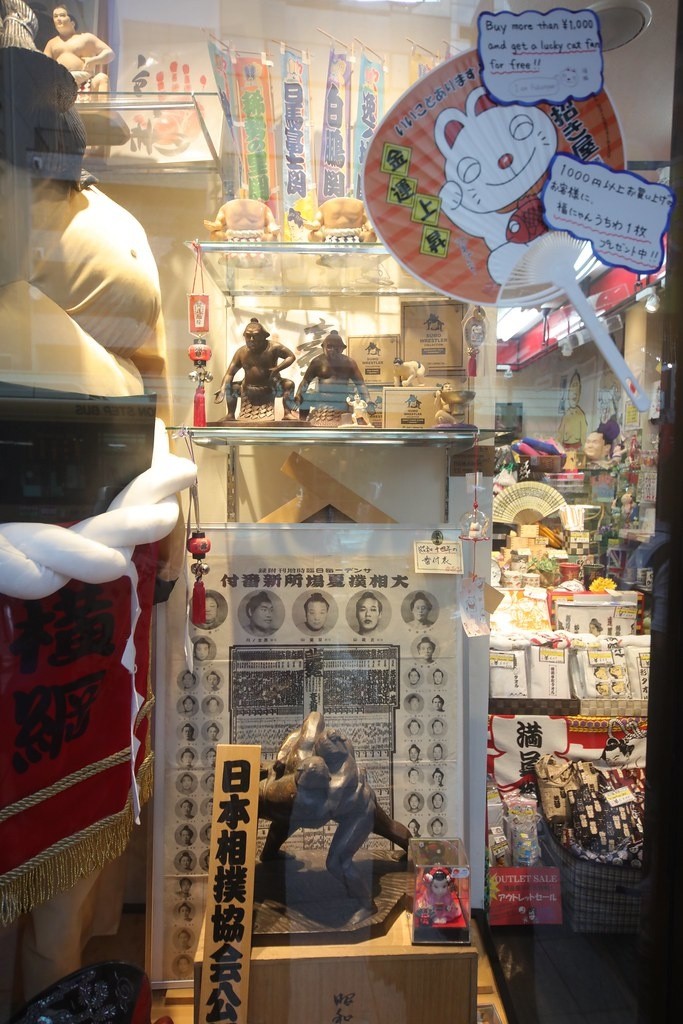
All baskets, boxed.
[519,453,567,473]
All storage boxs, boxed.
[487,839,564,936]
[542,821,643,937]
[517,454,562,473]
[496,523,594,577]
[547,586,645,637]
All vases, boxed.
[582,563,605,591]
[560,563,580,583]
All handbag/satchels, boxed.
[536,754,645,853]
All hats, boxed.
[0,46,87,182]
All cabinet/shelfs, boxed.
[160,241,516,450]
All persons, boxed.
[402,638,447,838]
[436,378,466,429]
[290,196,378,244]
[41,3,115,104]
[1,49,184,1019]
[393,358,428,387]
[418,869,463,926]
[298,593,330,635]
[243,588,279,638]
[584,419,644,526]
[177,635,221,975]
[406,590,434,629]
[203,194,279,258]
[295,331,374,427]
[214,317,292,421]
[355,591,383,635]
[199,593,220,625]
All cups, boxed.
[647,571,653,588]
[560,563,579,585]
[523,574,540,587]
[637,567,649,585]
[628,568,636,580]
[502,572,522,588]
[584,564,605,588]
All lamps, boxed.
[634,286,661,314]
[604,314,625,334]
[569,332,585,350]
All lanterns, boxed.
[188,339,212,367]
[186,532,211,559]
